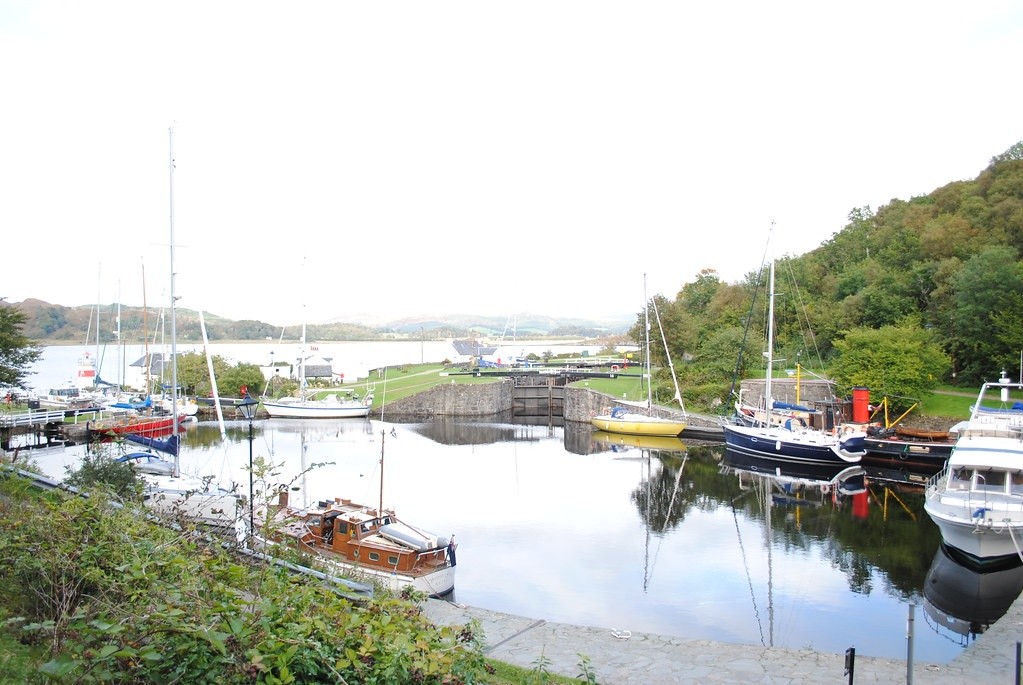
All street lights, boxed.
[235,390,257,551]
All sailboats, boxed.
[40,259,198,425]
[589,272,690,437]
[585,432,690,593]
[64,127,240,538]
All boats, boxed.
[920,542,1023,648]
[715,206,953,497]
[711,447,935,644]
[261,301,378,419]
[243,428,458,600]
[451,329,500,356]
[920,351,1023,563]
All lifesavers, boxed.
[95,422,103,429]
[769,417,776,424]
[899,451,909,461]
[843,426,855,435]
[900,467,908,475]
[819,485,830,494]
[777,418,783,425]
